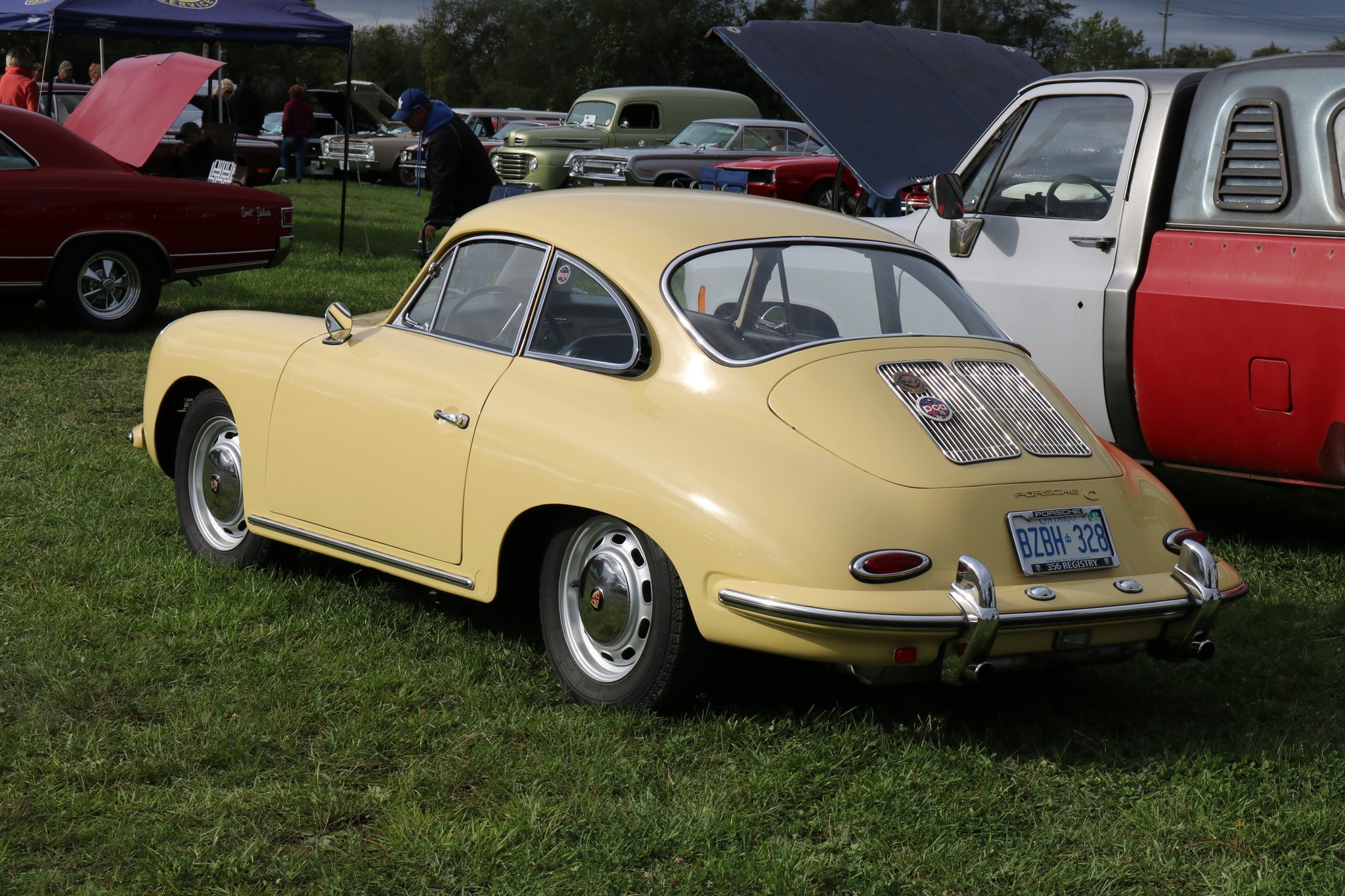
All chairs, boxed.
[672,166,750,194]
[411,186,534,269]
[556,333,650,371]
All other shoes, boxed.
[280,179,289,184]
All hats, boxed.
[391,88,430,122]
[31,63,43,71]
[59,60,73,70]
[175,121,199,140]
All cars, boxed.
[127,187,1250,710]
[0,51,296,337]
[561,119,931,217]
[34,79,570,191]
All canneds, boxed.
[418,239,428,252]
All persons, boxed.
[87,62,105,87]
[168,122,215,176]
[53,60,77,85]
[546,105,553,111]
[280,85,314,183]
[202,79,237,135]
[392,87,504,243]
[231,73,258,135]
[0,47,41,113]
[32,62,45,83]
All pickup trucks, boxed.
[682,20,1344,496]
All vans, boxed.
[489,87,790,190]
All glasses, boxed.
[223,89,233,95]
[179,133,193,142]
[37,69,43,72]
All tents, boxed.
[0,0,353,254]
